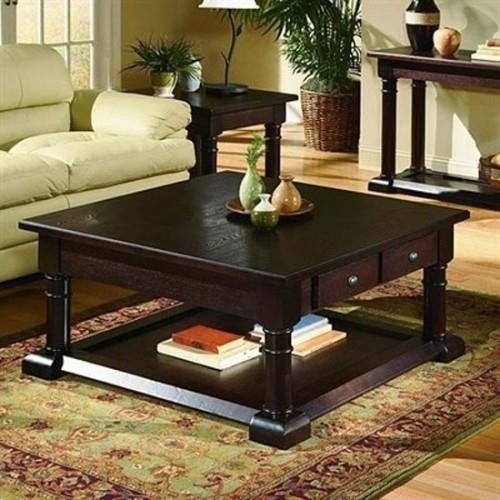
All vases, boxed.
[251,194,279,231]
[272,174,302,213]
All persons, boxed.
[291,339,346,361]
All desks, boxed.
[366,45,500,212]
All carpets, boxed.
[0,276,500,500]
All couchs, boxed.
[0,43,197,289]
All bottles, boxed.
[178,54,203,92]
[250,175,302,232]
[405,0,440,54]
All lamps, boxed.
[197,0,260,96]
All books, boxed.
[246,312,348,359]
[470,38,500,62]
[470,59,500,67]
[155,335,265,372]
[156,351,261,378]
[170,323,247,355]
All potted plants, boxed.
[239,135,269,211]
[117,32,207,97]
[215,0,362,153]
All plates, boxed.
[226,196,315,217]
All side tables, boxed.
[120,83,298,178]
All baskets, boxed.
[300,85,359,152]
[478,156,500,192]
